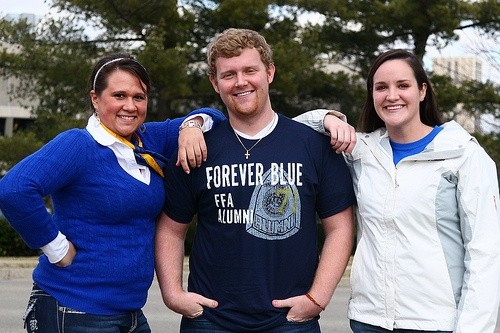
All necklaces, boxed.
[228,111,276,160]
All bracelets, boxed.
[178,120,202,133]
[305,293,325,312]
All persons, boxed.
[290,48,499,332]
[154,28,359,333]
[0,54,229,333]
